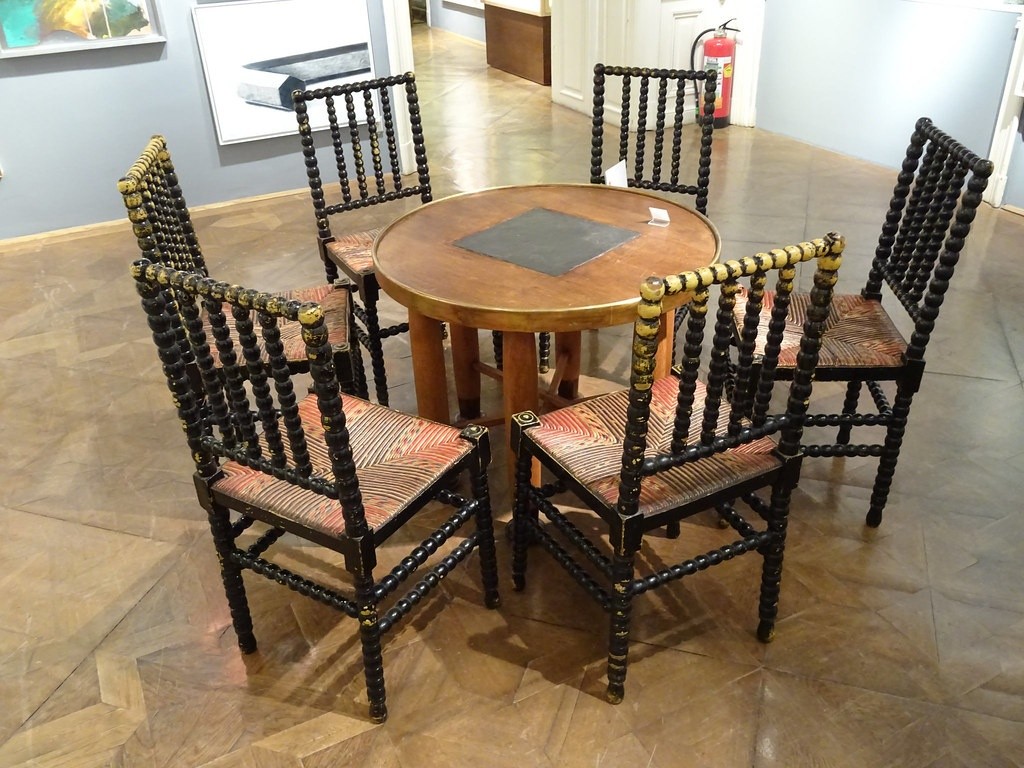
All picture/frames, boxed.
[191,0,380,146]
[0,0,167,59]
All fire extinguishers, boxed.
[689,17,741,130]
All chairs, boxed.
[507,232,844,705]
[132,258,500,724]
[719,119,994,528]
[539,64,718,373]
[293,71,505,410]
[118,136,370,420]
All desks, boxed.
[372,184,719,490]
